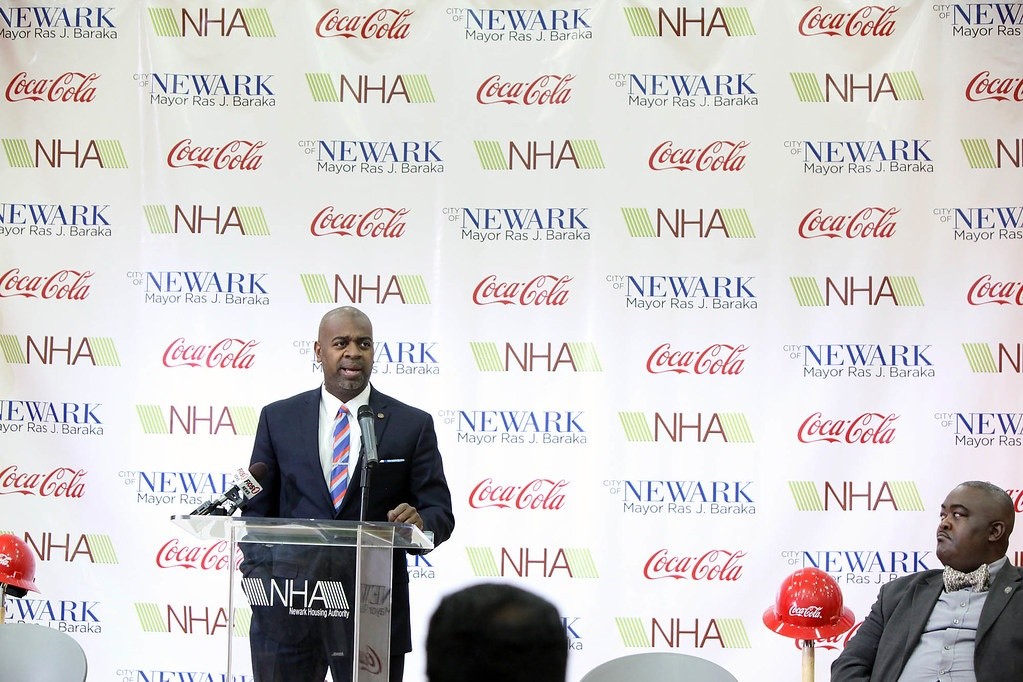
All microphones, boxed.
[357,404,378,467]
[190,463,269,515]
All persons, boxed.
[831,481,1023,682]
[425,583,568,682]
[237,307,455,682]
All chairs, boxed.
[0,622,88,682]
[581,652,738,682]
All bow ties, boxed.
[943,563,991,594]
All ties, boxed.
[329,405,350,512]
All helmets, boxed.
[0,534,42,598]
[762,567,856,640]
